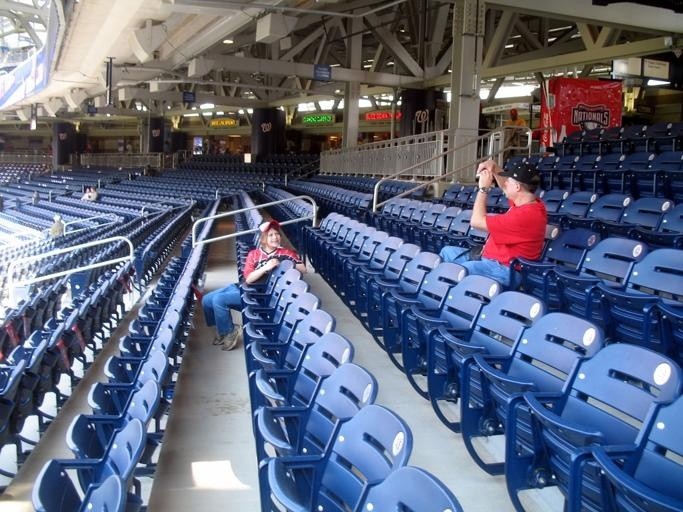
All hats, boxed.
[495,160,542,187]
[258,219,280,234]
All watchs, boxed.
[477,187,489,192]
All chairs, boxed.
[80,287,103,334]
[466,225,490,249]
[504,156,522,172]
[53,279,63,312]
[358,465,464,511]
[423,206,461,254]
[0,356,30,457]
[8,337,48,433]
[446,186,472,208]
[440,209,474,248]
[88,279,111,324]
[88,472,128,511]
[548,188,592,222]
[496,195,510,210]
[569,191,625,230]
[609,193,667,247]
[602,127,627,153]
[399,274,501,402]
[655,149,683,194]
[44,309,81,366]
[548,236,646,325]
[365,252,441,351]
[461,189,478,206]
[245,331,355,439]
[509,227,602,310]
[118,308,182,371]
[62,297,93,346]
[629,150,663,195]
[64,379,163,496]
[242,308,336,392]
[88,348,170,450]
[622,126,652,152]
[486,187,501,212]
[0,310,18,351]
[42,285,55,322]
[379,262,468,375]
[31,292,46,328]
[30,416,148,511]
[595,153,627,191]
[434,181,460,207]
[15,301,32,339]
[23,324,66,392]
[502,341,683,512]
[252,403,415,512]
[410,203,446,245]
[101,325,175,413]
[535,156,559,189]
[242,291,321,358]
[539,190,565,222]
[425,288,545,435]
[658,198,681,243]
[567,396,682,511]
[594,248,681,366]
[459,311,605,476]
[581,127,607,155]
[561,130,584,155]
[1,152,426,298]
[573,154,603,191]
[649,122,677,151]
[136,295,186,336]
[551,156,577,189]
[252,364,381,509]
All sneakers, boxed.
[212,333,226,346]
[222,322,244,352]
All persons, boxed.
[51,215,64,236]
[504,108,527,127]
[88,187,99,201]
[438,159,547,292]
[81,188,90,201]
[60,215,65,224]
[202,222,306,351]
